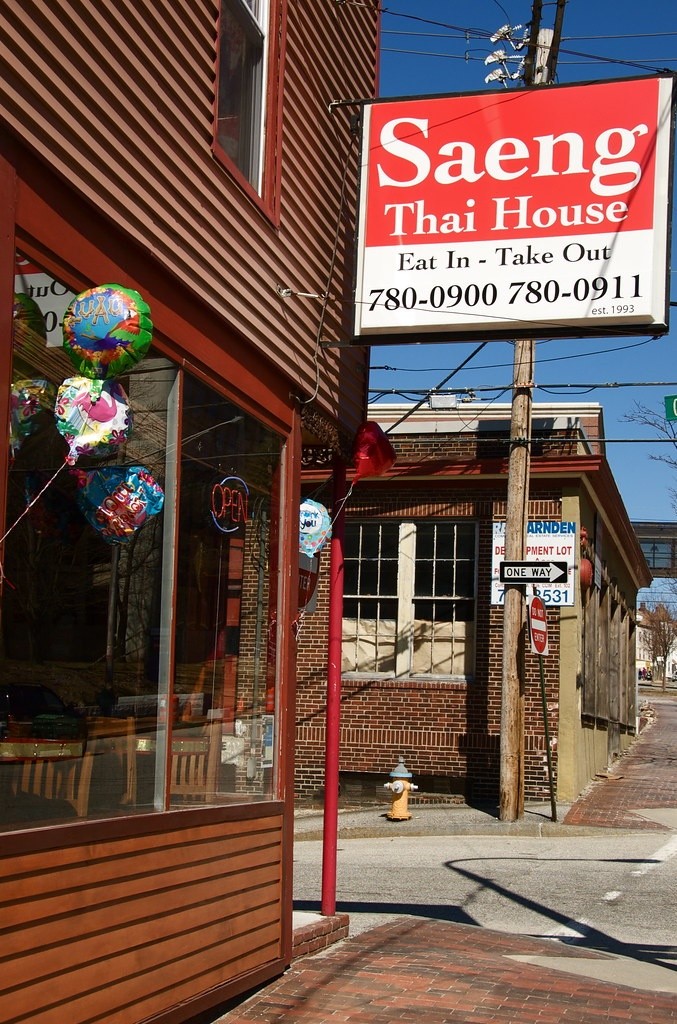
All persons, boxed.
[638,667,653,681]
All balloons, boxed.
[23,282,164,548]
[299,499,332,558]
[8,378,60,473]
[352,421,397,484]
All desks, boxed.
[105,737,207,756]
[0,736,82,762]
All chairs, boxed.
[169,718,222,810]
[83,715,154,819]
[6,717,98,821]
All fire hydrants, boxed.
[380,756,417,820]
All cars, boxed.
[672,671,677,682]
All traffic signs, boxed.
[500,560,571,586]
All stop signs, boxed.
[528,596,550,656]
